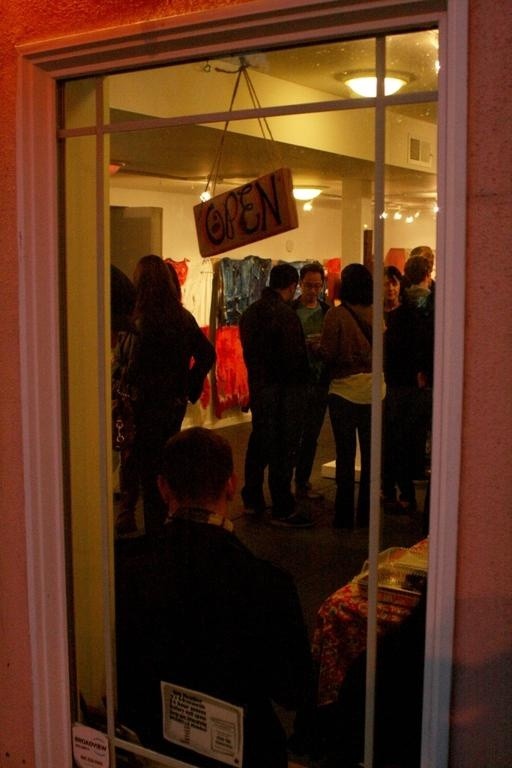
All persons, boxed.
[307,589,426,768]
[114,425,317,767]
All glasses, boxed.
[301,279,323,291]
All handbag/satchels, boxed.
[112,364,140,451]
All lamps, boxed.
[289,184,330,201]
[332,69,415,98]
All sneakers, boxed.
[329,514,369,533]
[268,507,323,529]
[383,500,420,516]
[294,483,327,499]
[243,503,269,518]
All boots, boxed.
[117,489,140,534]
